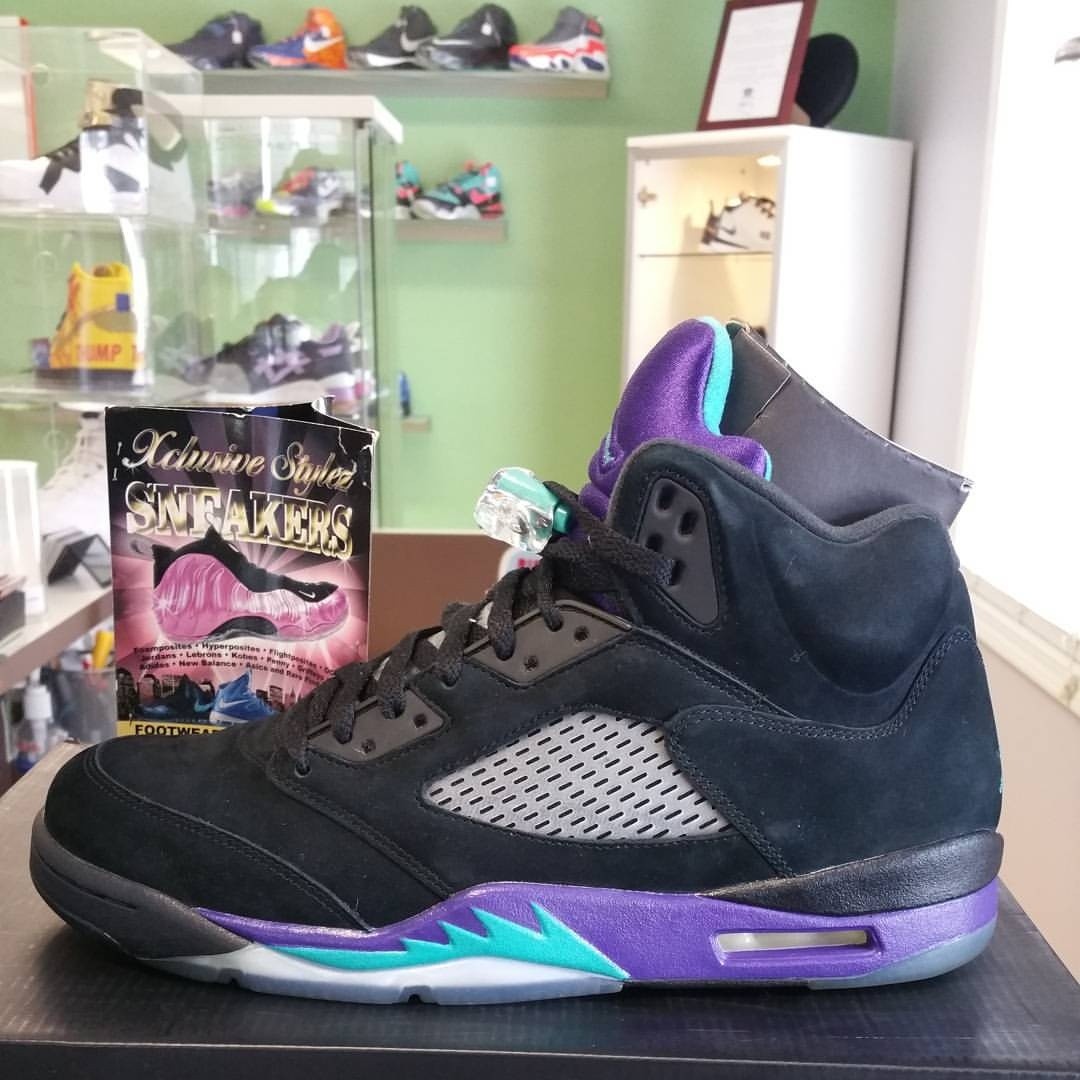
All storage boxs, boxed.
[0,738,1080,1080]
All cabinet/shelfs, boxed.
[146,69,615,246]
[620,125,914,441]
[0,24,405,431]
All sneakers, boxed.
[162,12,264,70]
[508,6,610,75]
[29,317,1005,1006]
[413,161,505,221]
[28,263,138,387]
[0,80,194,223]
[414,3,517,71]
[348,5,438,69]
[392,159,424,221]
[210,164,380,225]
[248,8,348,70]
[174,312,374,407]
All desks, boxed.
[0,575,113,696]
